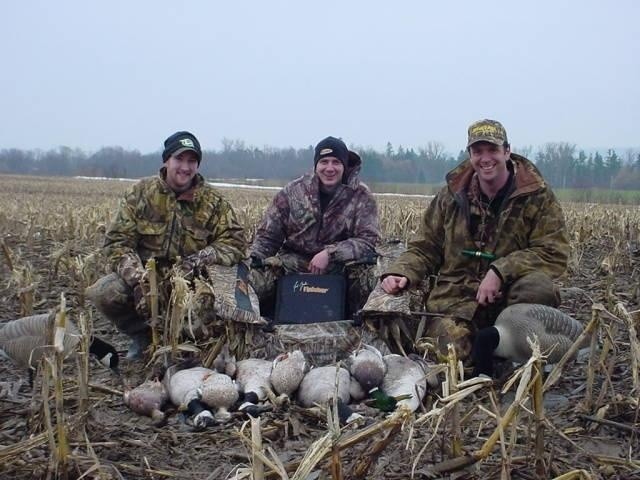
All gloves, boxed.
[182,259,195,282]
[132,283,150,316]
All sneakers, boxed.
[125,326,153,360]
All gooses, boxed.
[466,301,588,368]
[1,312,121,391]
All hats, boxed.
[465,118,508,149]
[313,135,349,172]
[162,131,202,167]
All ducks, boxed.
[120,341,440,431]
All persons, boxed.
[92,131,248,362]
[249,136,384,314]
[362,120,570,361]
